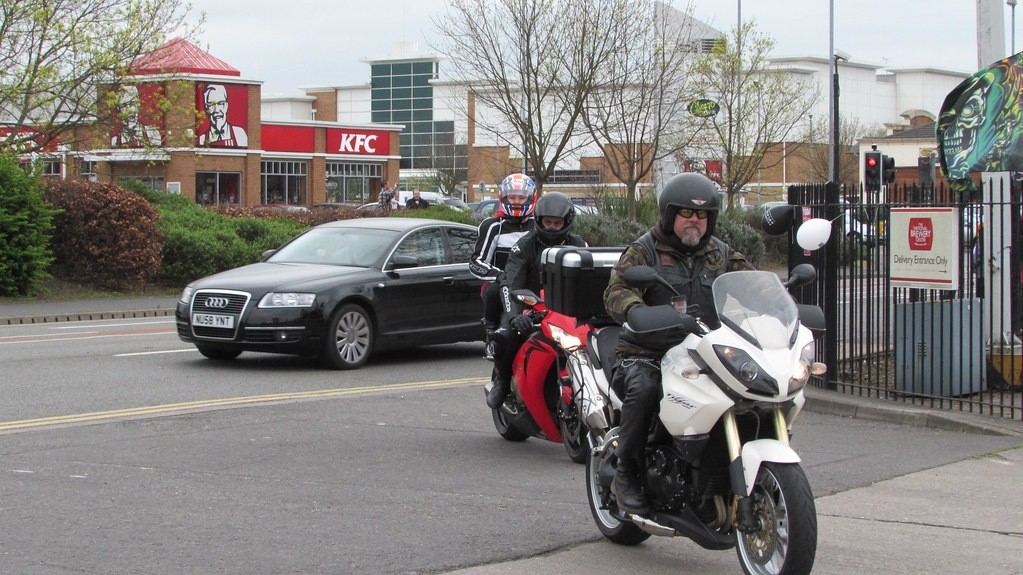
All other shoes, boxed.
[485,331,496,355]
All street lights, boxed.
[753,81,761,204]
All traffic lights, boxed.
[865,152,881,191]
[881,155,895,184]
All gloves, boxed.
[511,315,534,336]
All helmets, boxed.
[658,171,720,233]
[532,191,575,244]
[498,173,537,218]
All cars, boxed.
[839,200,886,245]
[468,201,599,217]
[176,214,480,370]
[964,206,982,272]
[356,193,367,200]
[255,203,384,211]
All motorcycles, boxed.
[481,280,601,465]
[540,243,827,575]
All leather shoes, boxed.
[609,460,652,513]
[486,374,511,409]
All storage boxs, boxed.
[540,245,628,324]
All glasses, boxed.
[677,209,709,219]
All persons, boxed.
[379,180,399,209]
[604,173,797,514]
[469,174,538,359]
[406,189,430,209]
[487,192,591,410]
[971,223,984,294]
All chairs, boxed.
[417,237,444,264]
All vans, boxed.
[399,191,472,212]
